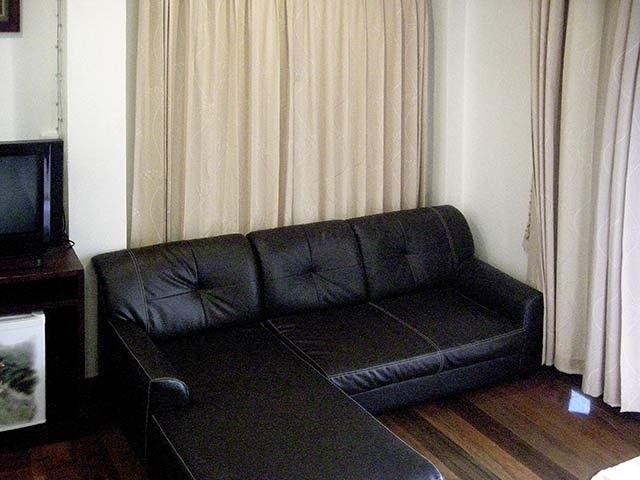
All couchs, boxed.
[246,205,544,409]
[91,232,444,478]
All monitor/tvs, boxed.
[0,139,64,260]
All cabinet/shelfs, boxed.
[0,242,88,449]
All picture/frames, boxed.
[0,0,24,38]
